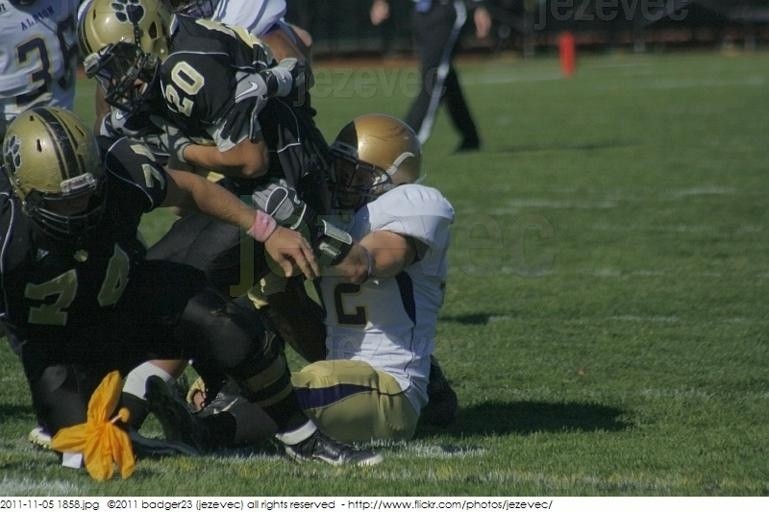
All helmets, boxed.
[326,114,423,214]
[78,0,171,119]
[3,106,106,241]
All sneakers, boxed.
[145,374,207,452]
[423,356,457,425]
[29,427,57,454]
[275,421,384,469]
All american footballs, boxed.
[302,167,332,244]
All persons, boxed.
[368,1,494,156]
[0,1,456,472]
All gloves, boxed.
[151,115,194,165]
[251,177,322,245]
[214,67,294,141]
